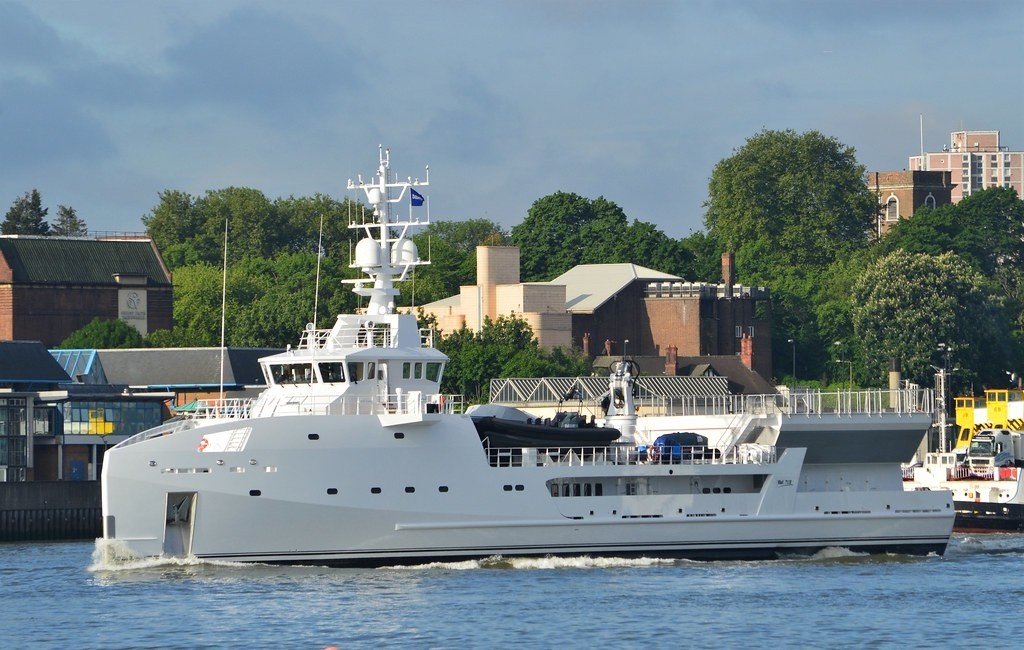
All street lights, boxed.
[937,342,953,418]
[836,359,852,392]
[787,338,795,407]
[834,341,846,394]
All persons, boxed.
[274,369,289,384]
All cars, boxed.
[902,462,924,479]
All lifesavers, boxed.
[1001,468,1006,479]
[650,446,659,460]
[1012,469,1017,477]
[431,394,444,413]
[1005,469,1010,478]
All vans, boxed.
[947,446,970,475]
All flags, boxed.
[410,188,425,206]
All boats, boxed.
[91,140,956,568]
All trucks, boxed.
[965,427,1024,480]
